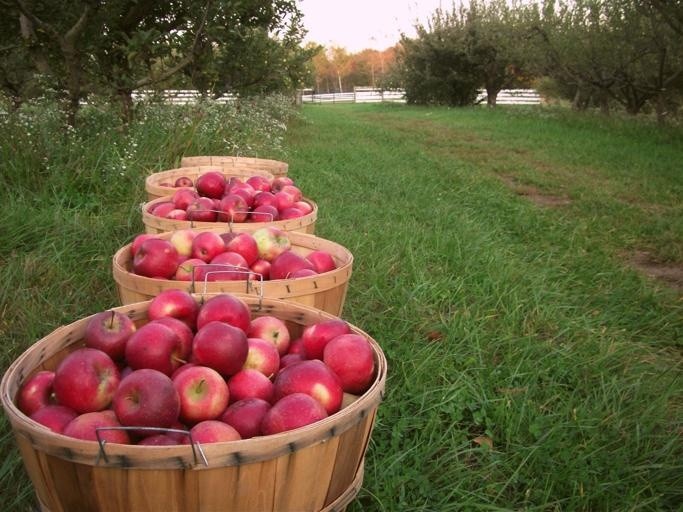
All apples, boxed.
[22,287,376,446]
[128,225,337,281]
[146,169,312,223]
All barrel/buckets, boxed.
[113,227,353,320]
[146,167,273,203]
[141,195,318,237]
[0,294,387,512]
[181,157,289,179]
